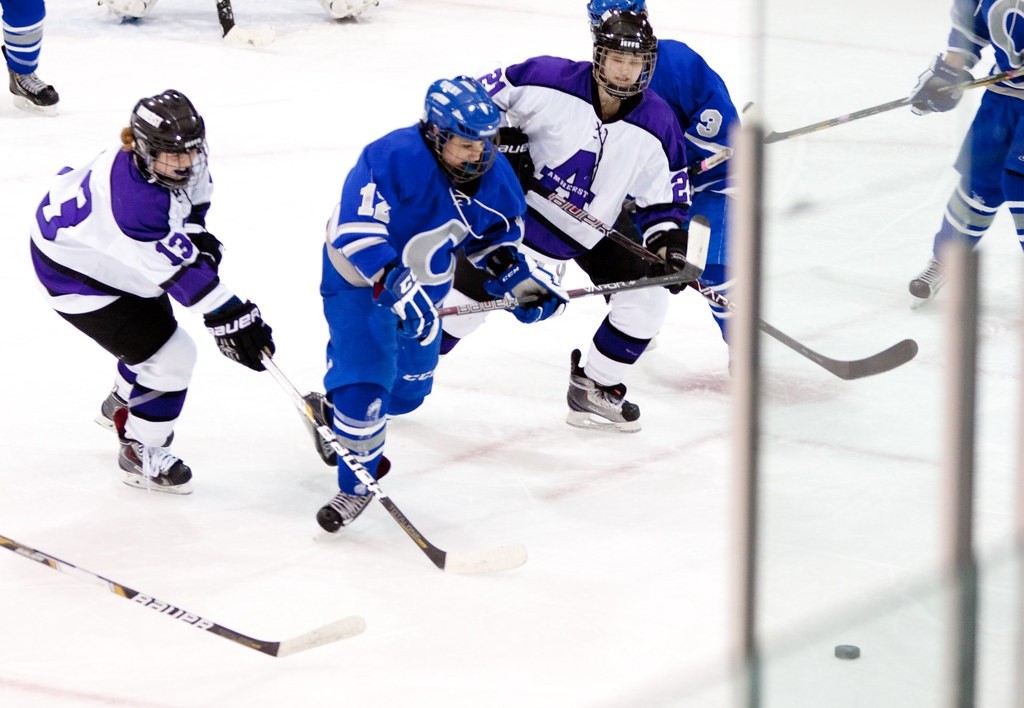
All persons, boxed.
[30,91,276,495]
[437,8,693,433]
[586,0,738,371]
[0,0,62,117]
[901,0,1024,311]
[315,73,572,535]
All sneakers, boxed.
[94,391,193,493]
[4,69,61,117]
[909,254,954,310]
[296,392,376,538]
[566,349,641,432]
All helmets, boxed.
[130,89,209,190]
[587,0,648,40]
[593,11,658,101]
[425,75,501,183]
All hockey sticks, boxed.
[1,533,371,662]
[743,67,1023,145]
[623,146,732,212]
[215,0,278,47]
[260,349,480,573]
[437,214,714,317]
[527,177,919,383]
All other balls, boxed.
[835,644,861,660]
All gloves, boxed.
[373,267,439,346]
[485,253,570,324]
[204,299,275,372]
[181,202,222,266]
[499,126,535,195]
[911,53,975,117]
[646,229,688,294]
